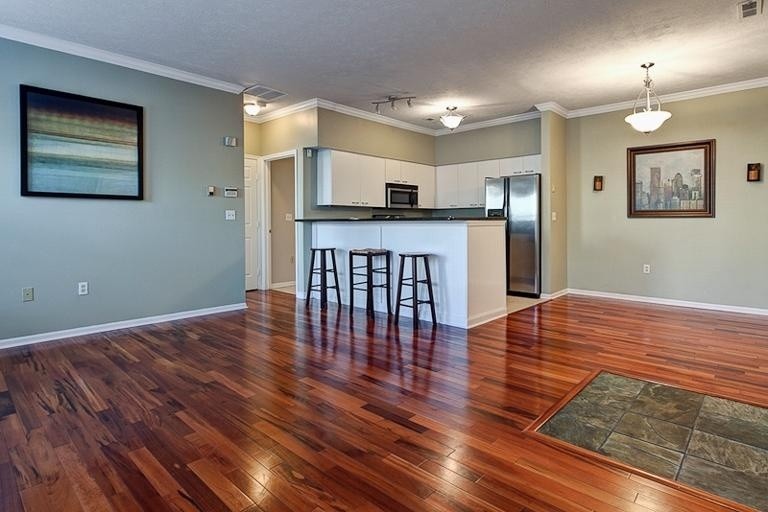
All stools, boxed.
[306,248,342,309]
[348,248,393,316]
[395,253,437,329]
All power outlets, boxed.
[79,281,89,295]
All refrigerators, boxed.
[485,173,541,298]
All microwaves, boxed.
[386,186,418,208]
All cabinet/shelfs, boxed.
[316,149,542,209]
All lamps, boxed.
[439,107,467,131]
[372,97,418,115]
[244,103,261,116]
[625,62,672,134]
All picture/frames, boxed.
[626,139,716,218]
[593,175,603,191]
[746,162,761,182]
[19,83,144,201]
